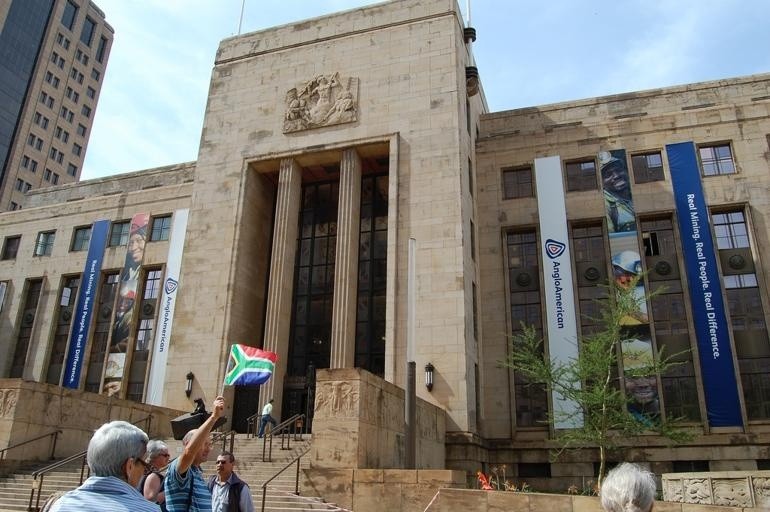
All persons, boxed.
[598,460,658,511]
[124,211,150,281]
[206,450,255,511]
[283,73,358,130]
[257,398,282,438]
[598,151,638,234]
[137,438,171,512]
[108,286,136,354]
[611,249,650,327]
[163,394,228,511]
[620,338,661,429]
[39,420,162,512]
[103,358,123,399]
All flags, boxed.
[224,344,279,385]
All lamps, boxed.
[425,362,434,392]
[185,371,194,398]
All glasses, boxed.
[135,457,151,470]
[160,453,170,457]
[215,459,229,464]
